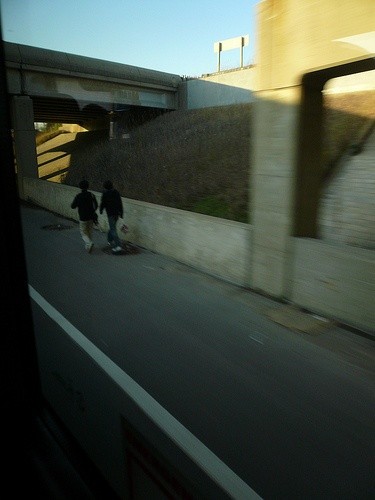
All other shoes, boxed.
[112,246,124,252]
[88,241,95,253]
[107,241,113,247]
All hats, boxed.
[74,180,90,188]
[102,180,114,189]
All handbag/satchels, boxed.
[93,212,99,225]
[120,215,128,233]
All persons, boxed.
[99,180,124,251]
[70,180,99,254]
